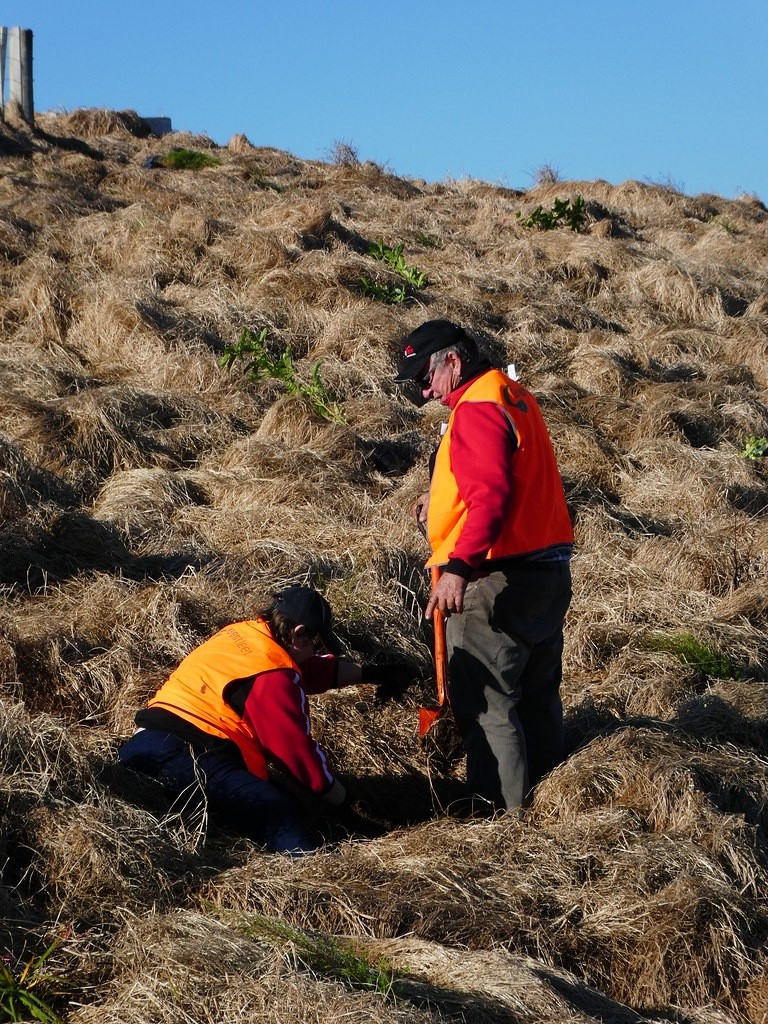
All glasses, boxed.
[414,363,438,388]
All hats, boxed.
[276,587,340,658]
[395,320,464,383]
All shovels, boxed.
[414,503,469,777]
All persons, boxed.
[118,585,411,863]
[393,321,572,813]
